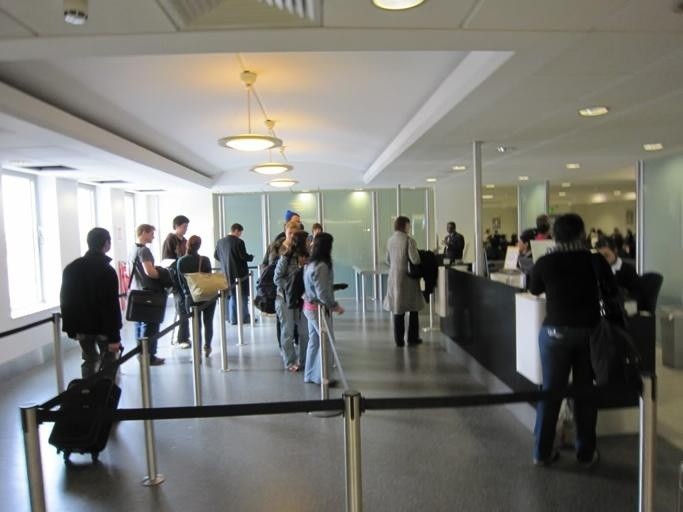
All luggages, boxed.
[48,377,121,468]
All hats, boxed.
[285,210,300,222]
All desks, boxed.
[353,264,393,311]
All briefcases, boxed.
[123,286,169,325]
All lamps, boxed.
[215,69,284,154]
[262,156,299,189]
[248,118,294,177]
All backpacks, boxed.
[282,265,304,310]
[253,263,279,315]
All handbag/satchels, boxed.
[184,270,230,303]
[587,322,645,385]
[405,258,426,279]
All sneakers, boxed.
[279,347,340,387]
[174,338,192,349]
[576,445,600,470]
[138,352,166,366]
[407,338,423,346]
[203,344,213,357]
[394,338,405,347]
[532,447,562,466]
[189,352,203,365]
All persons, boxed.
[60,228,122,378]
[484,214,643,304]
[443,222,464,257]
[527,216,623,468]
[383,217,425,346]
[126,212,344,373]
